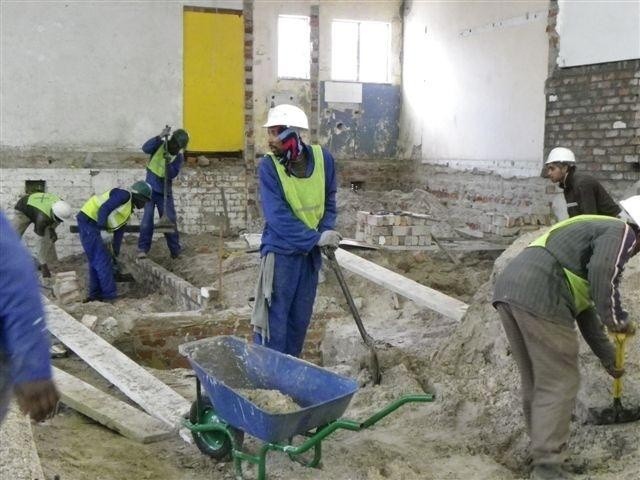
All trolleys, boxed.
[179,334,436,480]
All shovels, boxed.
[322,244,381,384]
[109,244,136,283]
[588,321,640,426]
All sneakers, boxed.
[138,252,147,257]
[116,288,126,297]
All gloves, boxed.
[603,362,626,378]
[619,318,638,337]
[49,227,57,242]
[159,126,171,139]
[15,377,60,425]
[40,263,52,278]
[316,230,343,249]
[162,151,172,166]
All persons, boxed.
[136,126,190,259]
[0,210,59,426]
[492,213,640,480]
[545,147,627,222]
[253,103,344,357]
[76,181,153,303]
[11,192,73,278]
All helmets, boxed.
[544,147,577,164]
[173,128,189,148]
[261,104,309,132]
[50,200,78,226]
[129,180,153,200]
[619,193,640,227]
